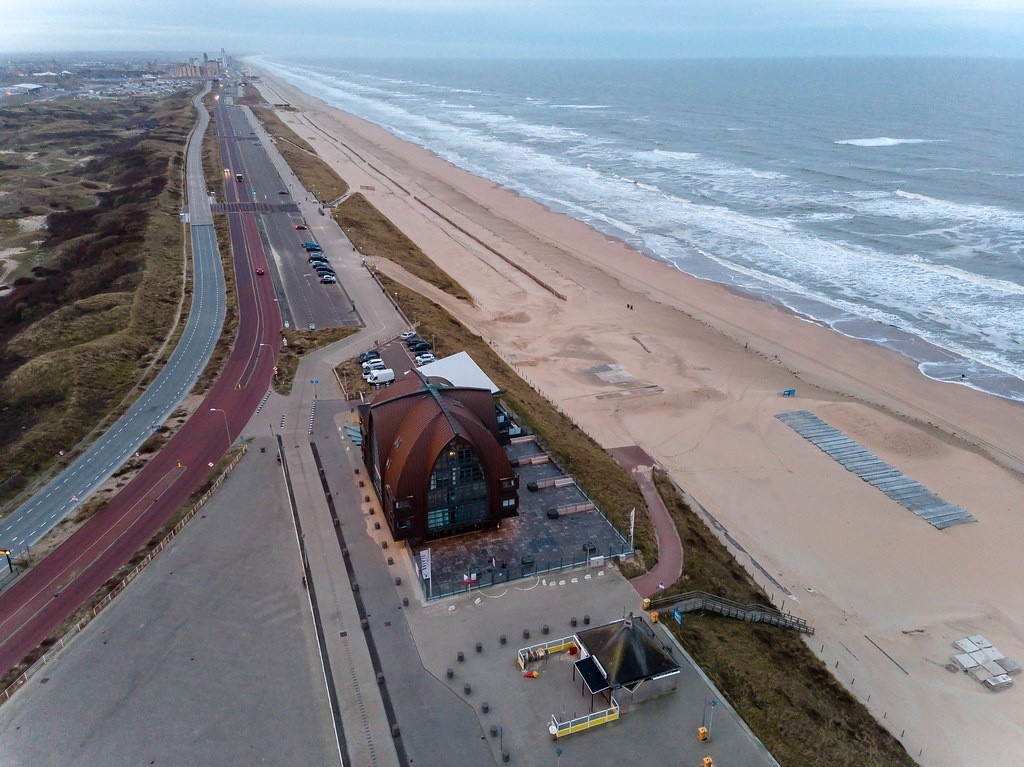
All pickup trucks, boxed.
[362,361,389,380]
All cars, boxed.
[256,266,265,275]
[406,337,427,347]
[400,330,417,341]
[295,224,308,229]
[278,189,289,194]
[417,358,435,365]
[301,241,337,284]
[358,351,381,364]
[414,350,437,356]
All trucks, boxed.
[366,368,395,386]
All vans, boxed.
[234,174,244,183]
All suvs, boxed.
[361,358,385,368]
[409,343,432,352]
[415,354,434,363]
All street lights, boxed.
[260,342,279,381]
[208,408,232,446]
